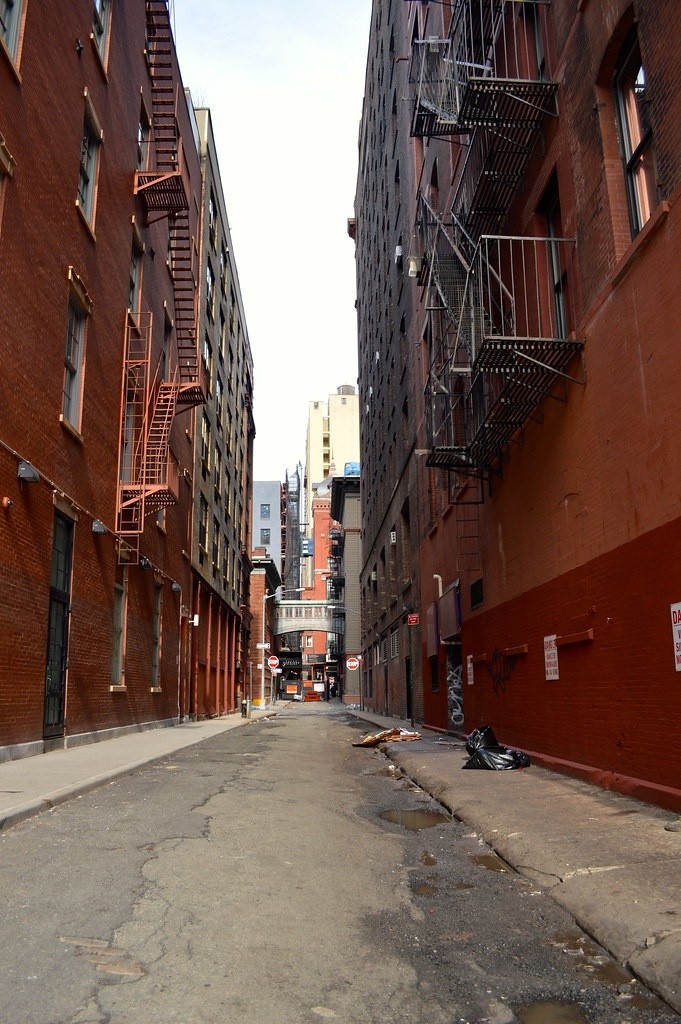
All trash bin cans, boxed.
[242,700,251,719]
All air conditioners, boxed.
[370,571,377,582]
[388,532,396,546]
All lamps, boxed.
[17,461,40,482]
[140,558,152,570]
[92,519,108,535]
[171,581,182,592]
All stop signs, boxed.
[329,678,335,684]
[346,658,359,670]
[267,656,279,669]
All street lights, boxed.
[327,605,361,616]
[259,588,306,712]
